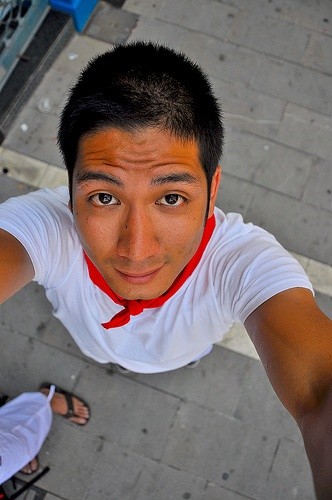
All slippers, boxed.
[18,454,39,476]
[38,381,91,425]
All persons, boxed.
[0,41,332,500]
[0,382,91,486]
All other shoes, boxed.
[117,365,130,374]
[185,358,201,369]
